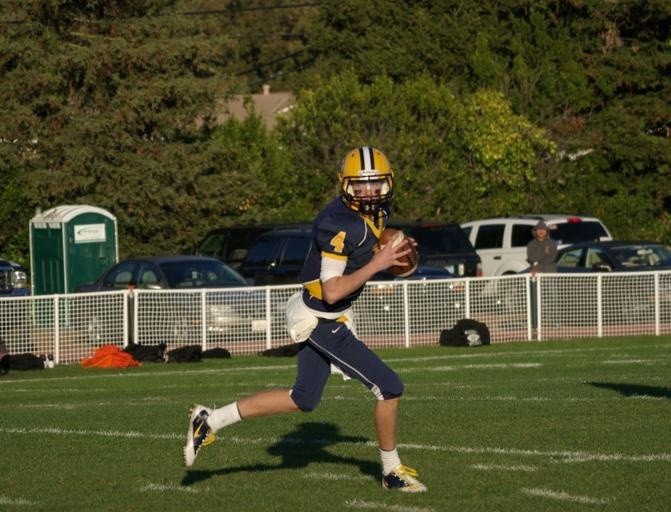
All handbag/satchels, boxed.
[122,342,230,363]
[440,319,490,346]
[0,353,44,374]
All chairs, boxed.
[560,252,605,267]
[118,271,157,285]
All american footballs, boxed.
[379,229,419,278]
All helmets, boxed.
[338,146,393,193]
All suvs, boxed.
[445,214,613,312]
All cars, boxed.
[183,220,490,331]
[71,256,279,346]
[0,258,29,297]
[553,241,671,322]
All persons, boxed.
[527,221,559,272]
[183,146,427,495]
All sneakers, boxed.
[183,403,216,467]
[381,464,427,493]
